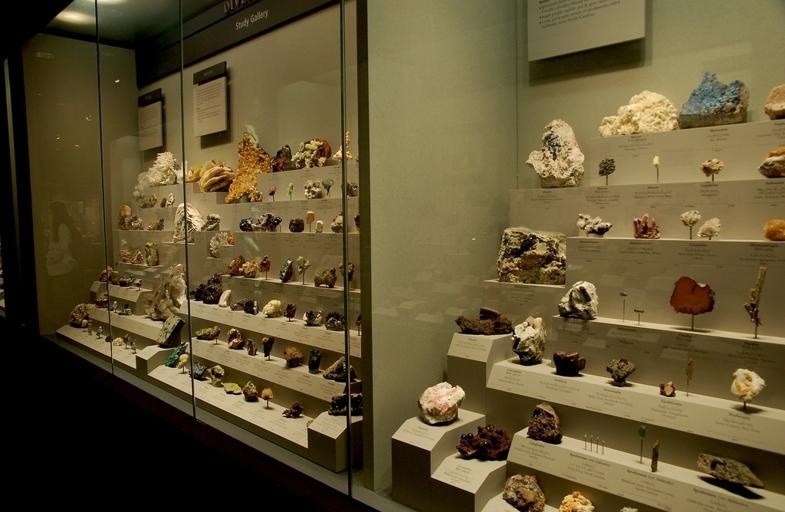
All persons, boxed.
[44,200,81,334]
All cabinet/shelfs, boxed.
[386,117,785,511]
[57,153,361,471]
